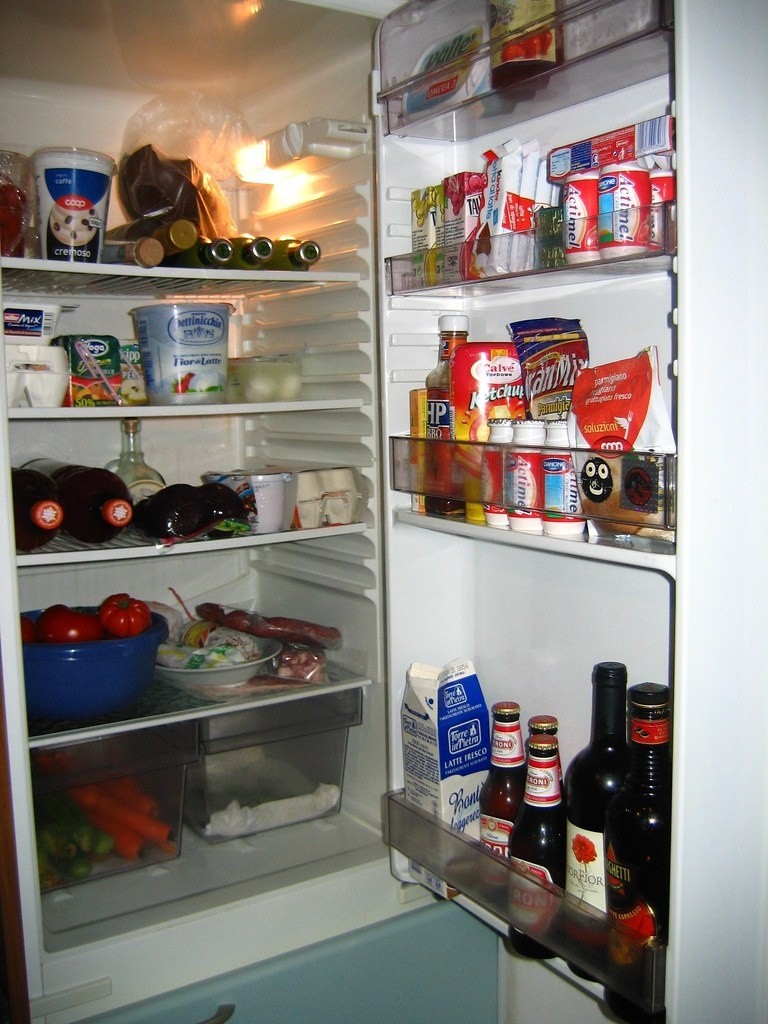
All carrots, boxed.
[38,748,175,860]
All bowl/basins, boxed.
[158,641,283,689]
[22,607,169,719]
[251,474,287,534]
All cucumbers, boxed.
[30,758,114,888]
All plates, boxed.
[264,239,321,270]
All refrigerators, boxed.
[0,0,768,1024]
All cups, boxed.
[0,150,46,260]
[31,148,119,263]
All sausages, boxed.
[195,602,342,650]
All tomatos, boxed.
[22,592,151,644]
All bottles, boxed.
[602,682,672,1024]
[158,237,235,269]
[542,419,585,535]
[228,236,272,269]
[19,457,133,543]
[482,418,515,525]
[100,237,164,269]
[527,714,563,787]
[481,703,526,902]
[425,315,468,518]
[105,419,166,505]
[507,421,546,531]
[564,662,627,983]
[509,736,564,960]
[11,468,63,550]
[106,219,198,257]
